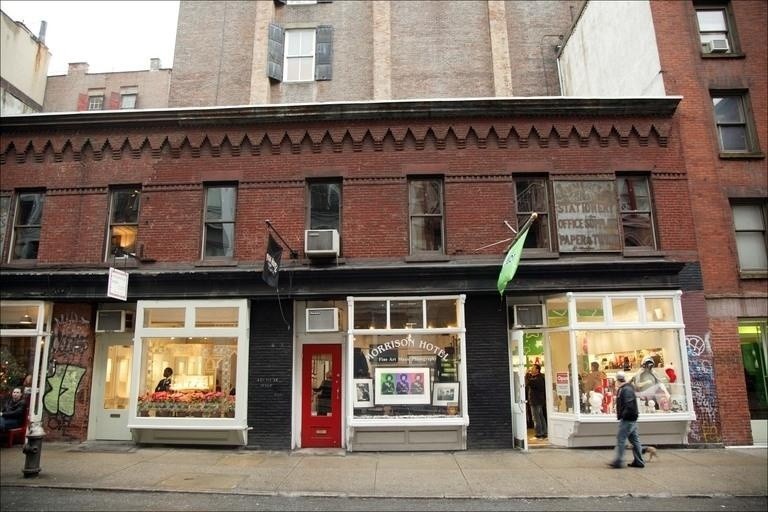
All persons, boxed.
[437,390,454,400]
[357,384,368,401]
[410,374,424,394]
[526,363,547,438]
[606,371,646,468]
[0,386,25,432]
[313,371,331,416]
[566,355,631,413]
[154,366,174,393]
[382,374,395,394]
[396,374,409,394]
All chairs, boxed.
[4,407,30,449]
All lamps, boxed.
[18,306,32,325]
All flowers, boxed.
[137,390,236,414]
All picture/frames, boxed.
[351,366,462,409]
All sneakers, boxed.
[604,462,644,469]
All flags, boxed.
[495,221,533,297]
[260,232,284,288]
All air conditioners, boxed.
[509,301,548,331]
[304,305,344,332]
[304,228,341,261]
[708,36,732,55]
[94,309,134,334]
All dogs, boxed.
[627,442,660,462]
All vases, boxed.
[139,410,234,418]
